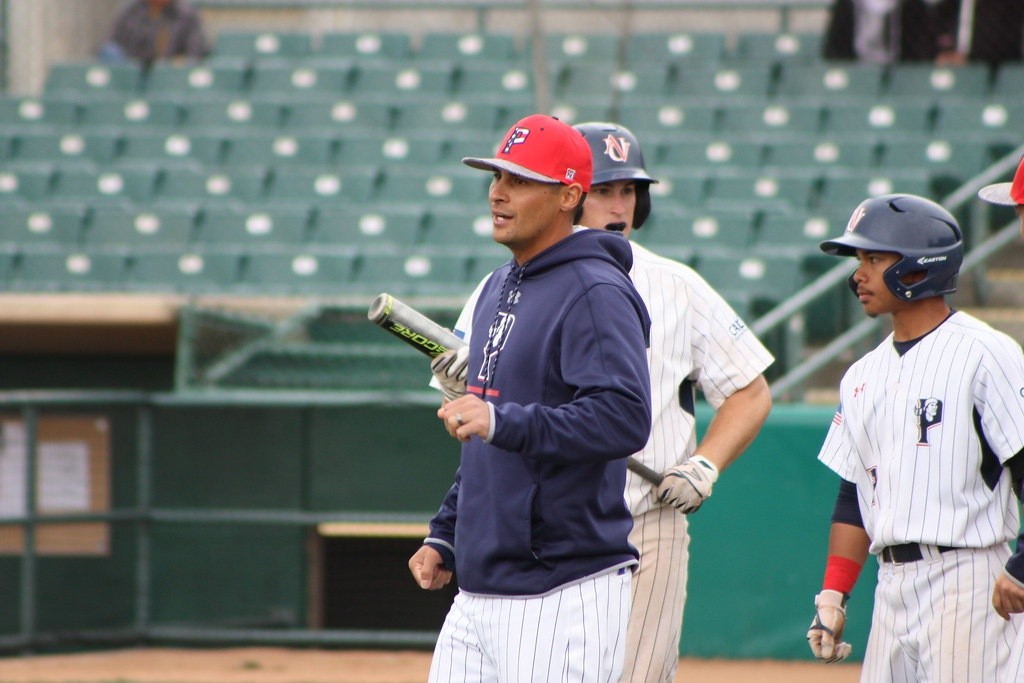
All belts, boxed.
[882,542,965,566]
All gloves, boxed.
[806,590,852,665]
[650,455,720,514]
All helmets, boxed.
[820,192,964,318]
[572,121,660,184]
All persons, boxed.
[408,113,652,683]
[823,1,936,65]
[430,122,775,683]
[94,0,208,75]
[806,194,1024,683]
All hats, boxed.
[977,154,1024,206]
[461,113,593,193]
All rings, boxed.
[455,412,464,425]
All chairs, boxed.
[0,29,1024,402]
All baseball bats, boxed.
[366,292,702,513]
